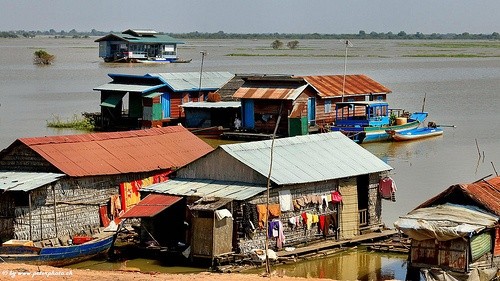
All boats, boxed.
[136,56,170,64]
[171,59,192,63]
[0,220,122,265]
[385,126,444,139]
[326,102,428,143]
